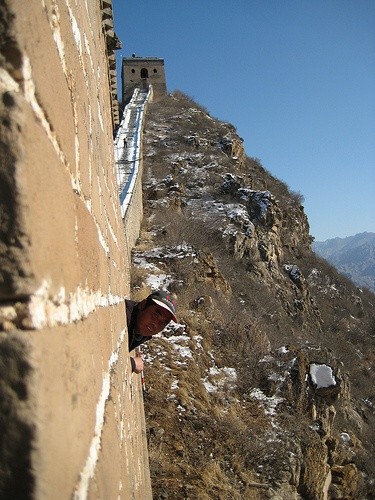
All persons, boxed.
[124,289,179,375]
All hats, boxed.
[147,290,177,324]
